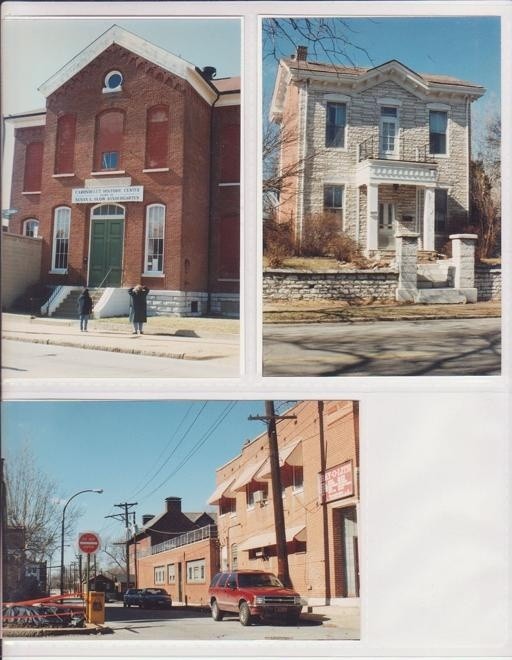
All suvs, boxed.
[208,569,308,627]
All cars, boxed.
[137,587,172,609]
[123,589,143,606]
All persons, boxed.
[128,284,150,334]
[77,288,93,332]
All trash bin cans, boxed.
[89,592,105,624]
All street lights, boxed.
[59,489,103,603]
[49,544,70,588]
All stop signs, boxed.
[78,532,100,553]
